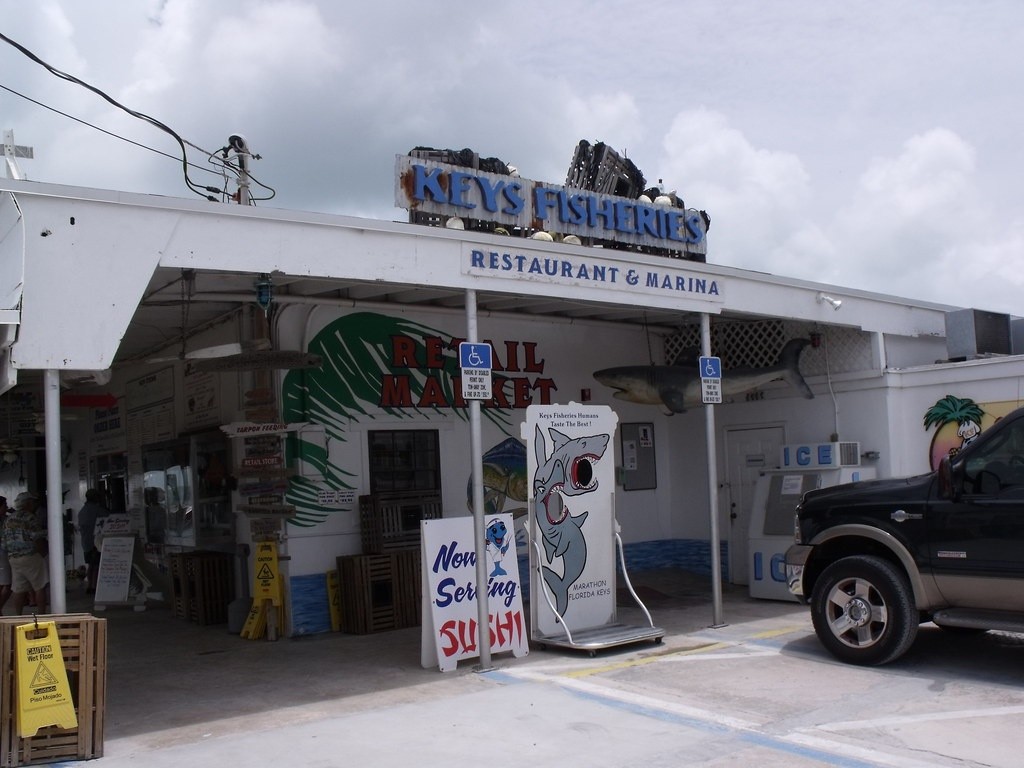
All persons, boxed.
[1,492,49,615]
[78,488,111,595]
[0,495,11,616]
[143,486,170,544]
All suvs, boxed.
[780,405,1024,668]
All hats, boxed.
[13,492,37,508]
